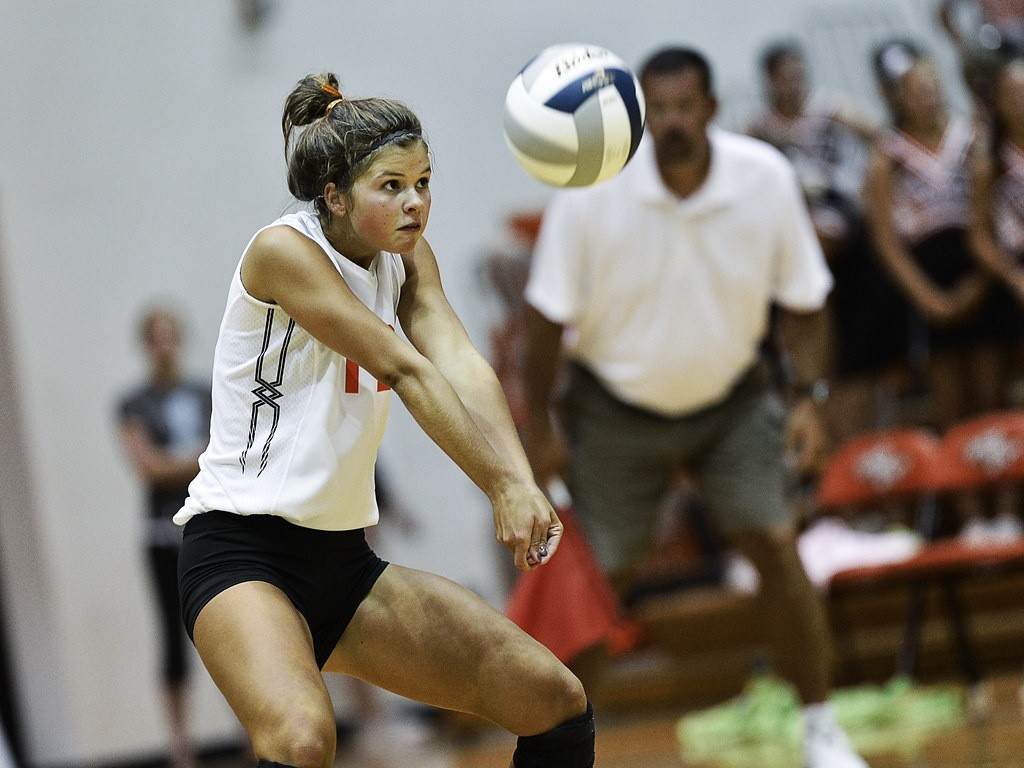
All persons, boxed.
[173,75,596,768]
[524,48,872,768]
[489,0,1024,585]
[114,309,212,768]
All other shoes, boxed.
[795,703,870,768]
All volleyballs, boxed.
[499,36,648,189]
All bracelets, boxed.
[794,380,830,403]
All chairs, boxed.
[488,166,1024,723]
